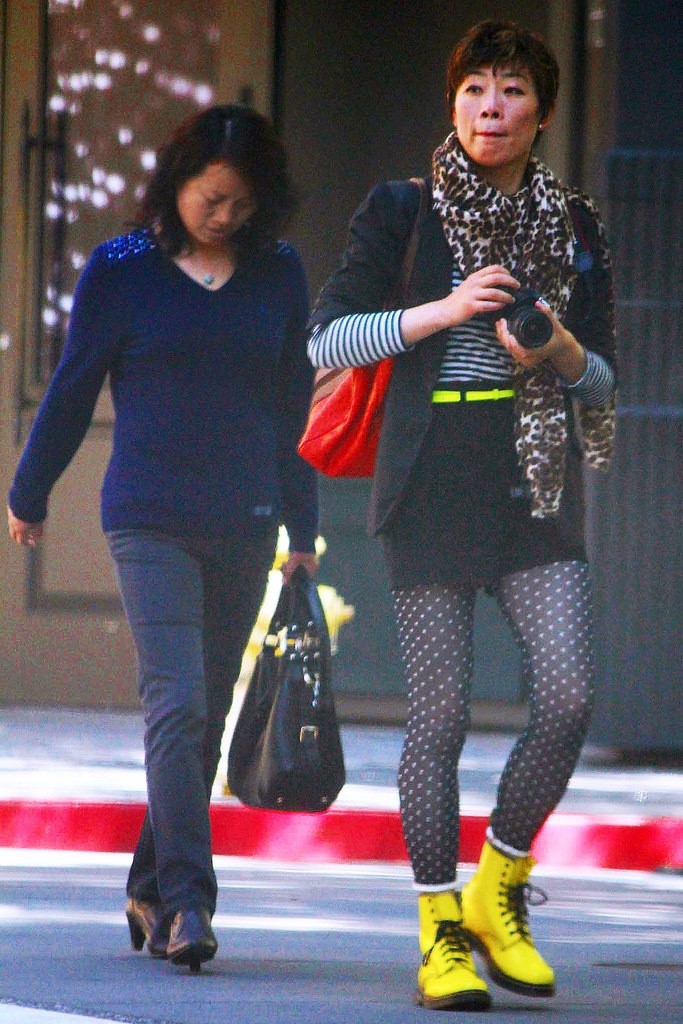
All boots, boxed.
[413,888,490,1008]
[460,838,556,997]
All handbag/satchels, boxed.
[227,567,347,815]
[296,178,429,479]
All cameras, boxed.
[475,284,554,349]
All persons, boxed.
[305,15,620,1010]
[8,104,321,973]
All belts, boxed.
[432,389,516,402]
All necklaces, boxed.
[180,238,234,287]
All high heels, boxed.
[124,897,166,956]
[168,905,218,971]
